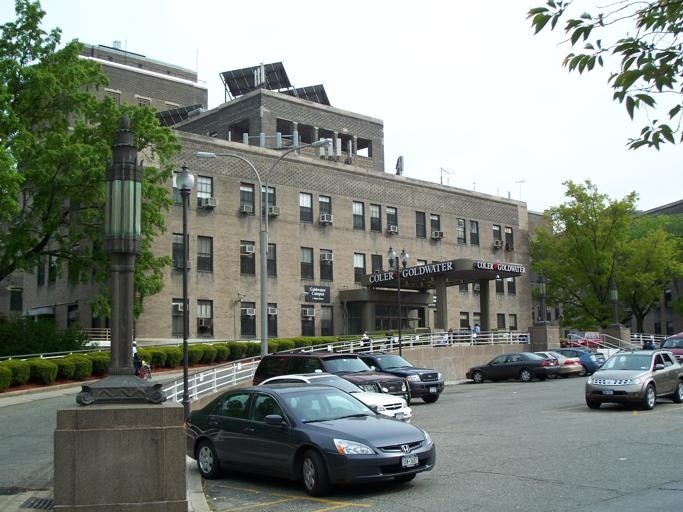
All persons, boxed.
[471,324,480,344]
[385,327,394,348]
[360,333,369,351]
[132,341,140,375]
[649,340,655,350]
[444,328,455,343]
[642,340,652,349]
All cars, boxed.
[465,331,682,411]
[184,351,446,497]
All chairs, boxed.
[221,398,243,418]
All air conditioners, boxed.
[239,242,256,256]
[493,239,514,250]
[319,252,334,263]
[238,303,315,321]
[320,213,333,223]
[201,196,218,209]
[431,230,445,241]
[240,203,281,217]
[387,224,399,234]
[174,301,213,332]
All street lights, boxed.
[196,138,334,360]
[386,243,409,357]
[176,160,195,418]
